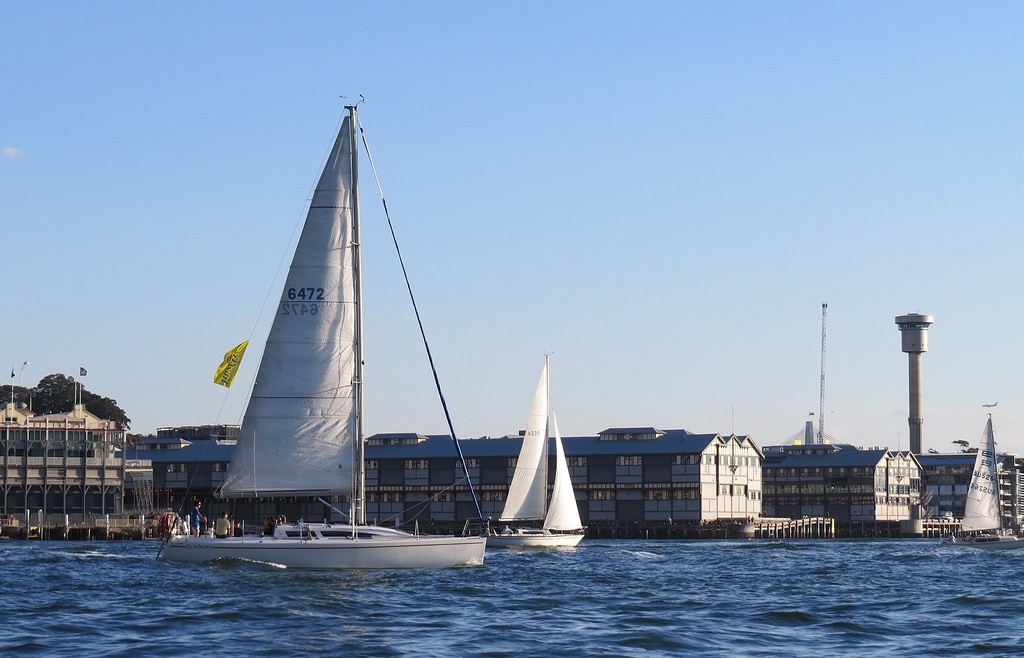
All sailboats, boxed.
[157,92,490,570]
[937,412,1023,551]
[477,355,589,549]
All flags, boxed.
[809,412,815,415]
[213,339,250,389]
[80,366,87,376]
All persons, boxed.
[191,499,207,538]
[263,513,286,535]
[215,511,243,539]
[502,524,513,534]
